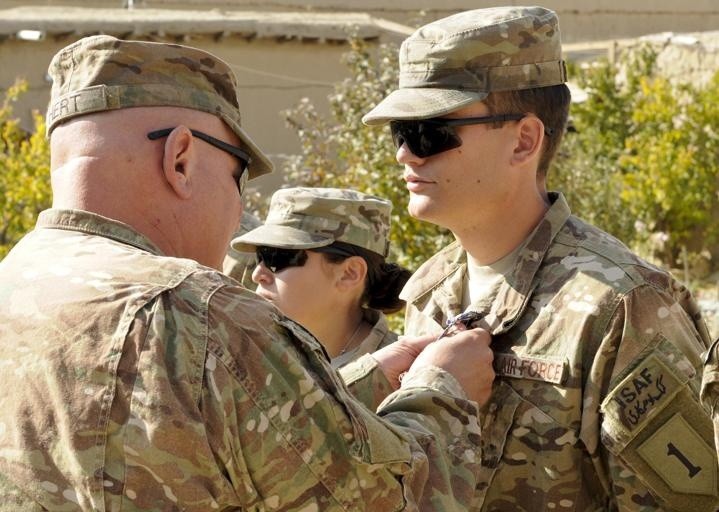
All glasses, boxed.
[147,127,253,196]
[256,246,351,273]
[390,114,555,158]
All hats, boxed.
[46,34,276,181]
[230,186,393,258]
[361,6,568,126]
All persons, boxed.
[361,6,719,512]
[0,34,496,512]
[230,186,412,368]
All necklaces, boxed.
[340,322,362,355]
